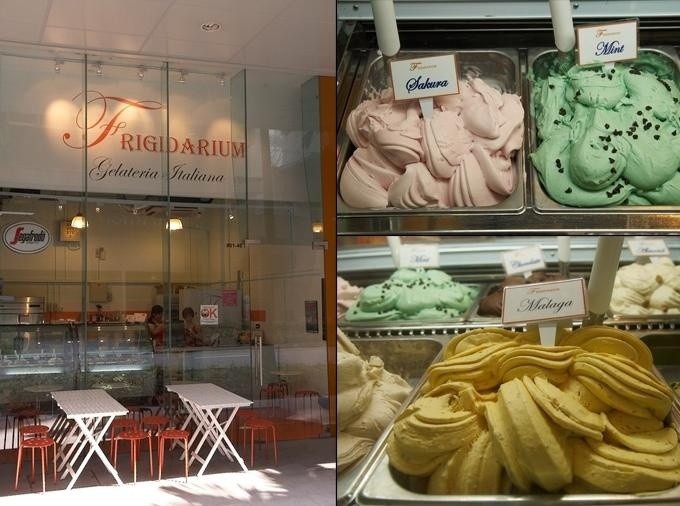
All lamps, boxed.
[70,202,89,229]
[165,212,183,231]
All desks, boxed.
[50,388,130,490]
[285,406,336,426]
[165,382,254,478]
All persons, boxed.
[182,308,202,347]
[148,305,166,346]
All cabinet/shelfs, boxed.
[76,322,157,405]
[0,321,79,413]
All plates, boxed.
[340,273,482,325]
[525,39,680,218]
[338,43,527,221]
[579,265,680,325]
[339,277,365,323]
[467,272,580,323]
[339,334,450,504]
[357,325,676,506]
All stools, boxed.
[259,381,290,430]
[142,416,172,465]
[14,438,56,495]
[114,429,154,486]
[18,425,49,483]
[139,407,153,432]
[295,389,323,439]
[158,430,190,484]
[242,419,278,468]
[235,409,265,452]
[4,408,41,457]
[110,418,140,465]
[128,407,141,430]
